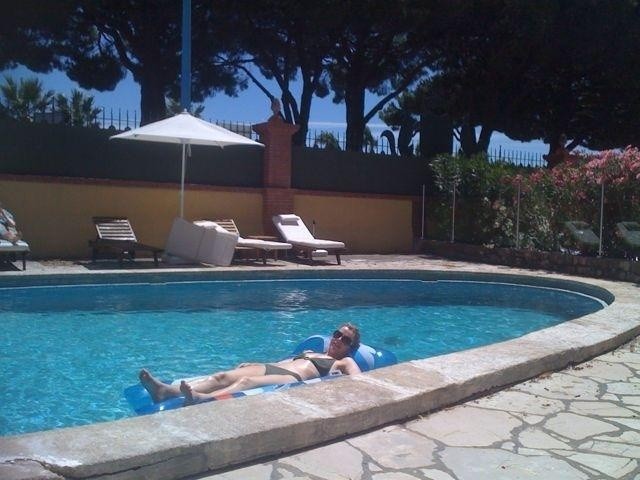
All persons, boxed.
[139,321,362,406]
[0,205,23,243]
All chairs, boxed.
[0,208,32,271]
[193,217,293,266]
[89,216,164,267]
[271,213,347,265]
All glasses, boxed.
[333,330,353,345]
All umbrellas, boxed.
[109,107,267,215]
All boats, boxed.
[124,335,397,416]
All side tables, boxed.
[247,235,280,261]
